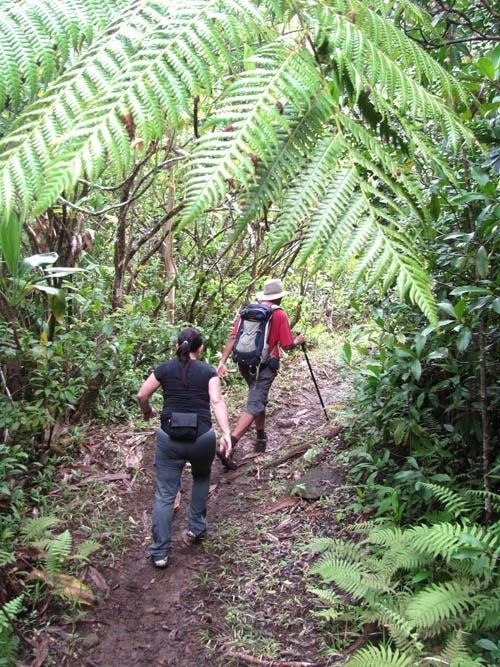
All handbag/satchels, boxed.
[169,411,197,443]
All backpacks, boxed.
[232,305,282,366]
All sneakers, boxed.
[216,446,237,469]
[154,556,168,567]
[256,436,267,452]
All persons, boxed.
[137,328,232,568]
[215,279,305,470]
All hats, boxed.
[255,279,291,300]
